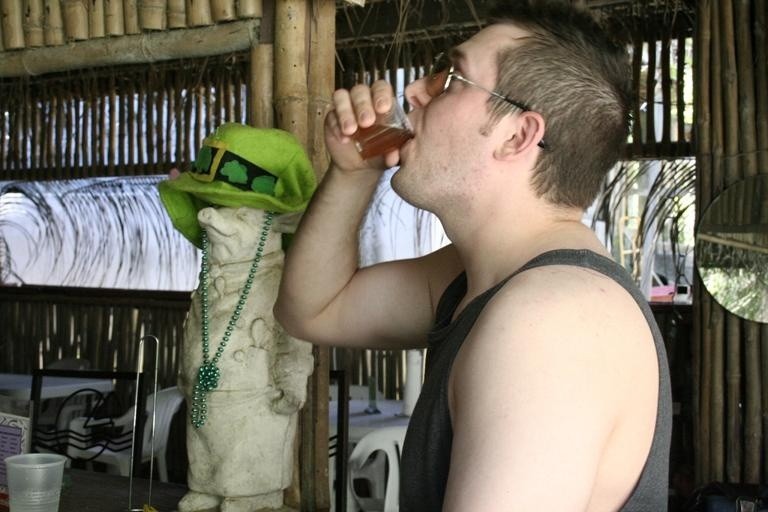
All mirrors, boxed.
[693,173,767,328]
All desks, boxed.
[1,371,116,452]
[328,397,410,496]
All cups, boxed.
[3,452,69,512]
[352,101,414,160]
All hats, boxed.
[157,121,316,251]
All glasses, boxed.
[425,51,547,149]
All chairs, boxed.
[30,366,185,485]
[350,426,408,511]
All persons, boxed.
[272,1,675,512]
